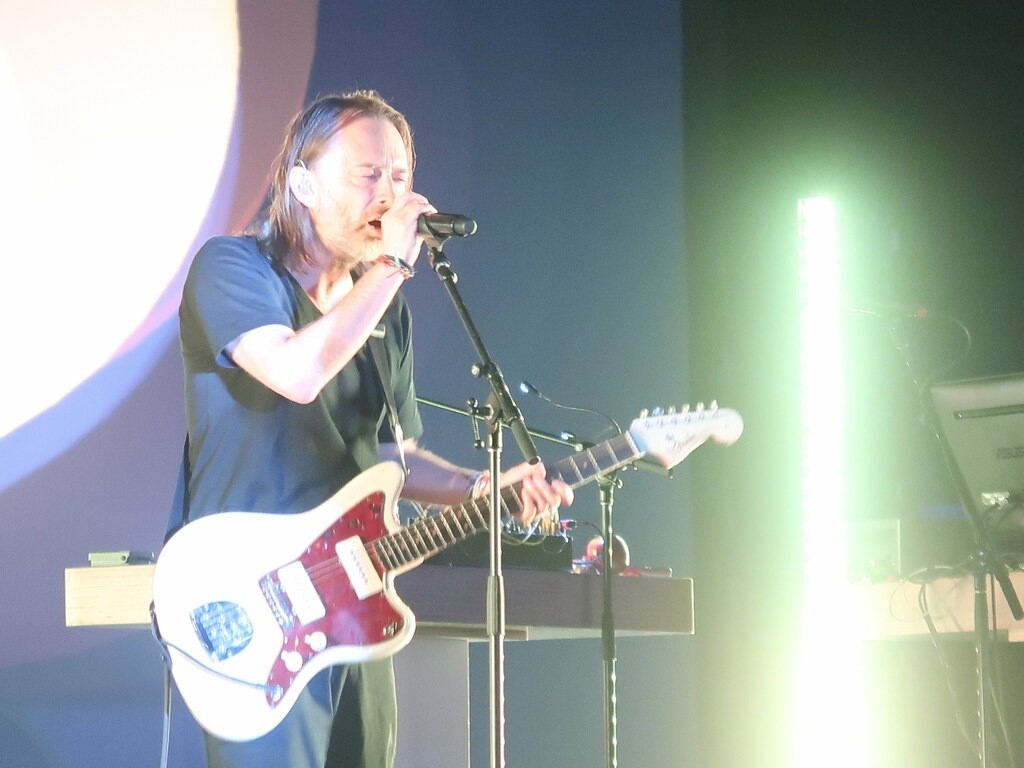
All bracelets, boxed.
[466,470,501,499]
[373,254,416,281]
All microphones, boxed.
[417,213,478,241]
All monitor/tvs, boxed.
[892,368,1024,578]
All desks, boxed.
[875,570,1024,643]
[65,568,695,768]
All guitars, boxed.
[150,398,745,744]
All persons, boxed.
[160,92,575,768]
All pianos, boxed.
[63,564,695,639]
